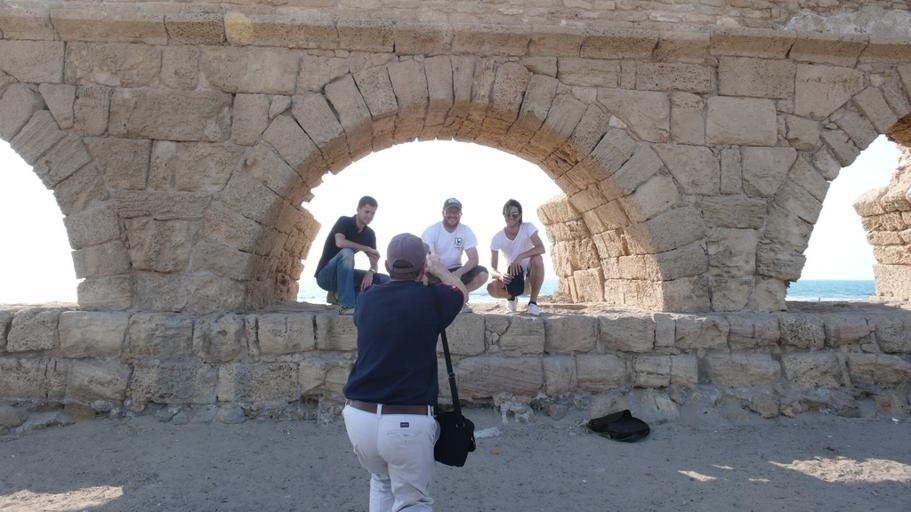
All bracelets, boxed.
[369,268,377,275]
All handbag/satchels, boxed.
[587,409,651,441]
[431,409,476,467]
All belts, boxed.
[349,399,434,415]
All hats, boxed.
[386,233,432,274]
[507,264,525,296]
[443,197,463,212]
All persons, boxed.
[487,199,547,315]
[314,195,392,314]
[420,197,491,314]
[342,233,472,512]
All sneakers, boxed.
[339,303,356,315]
[527,302,539,316]
[327,290,339,304]
[507,296,518,312]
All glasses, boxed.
[505,212,521,219]
